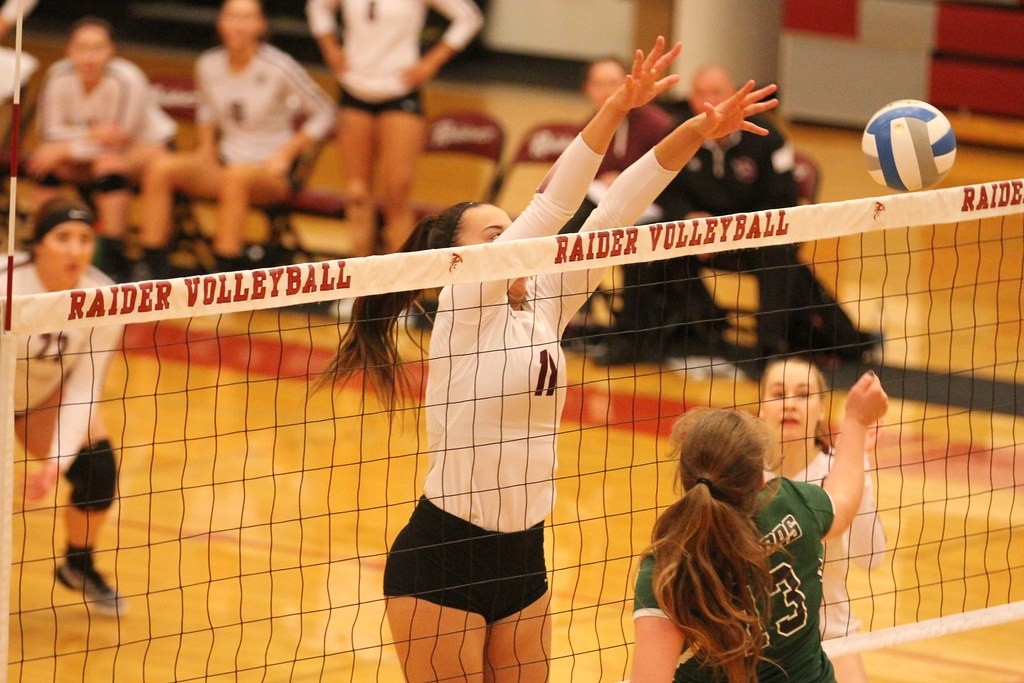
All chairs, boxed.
[16,73,833,375]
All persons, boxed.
[0,201,126,612]
[562,54,806,368]
[628,357,890,683]
[0,0,487,326]
[379,36,775,683]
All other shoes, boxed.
[56,560,125,615]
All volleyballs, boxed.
[859,99,958,192]
